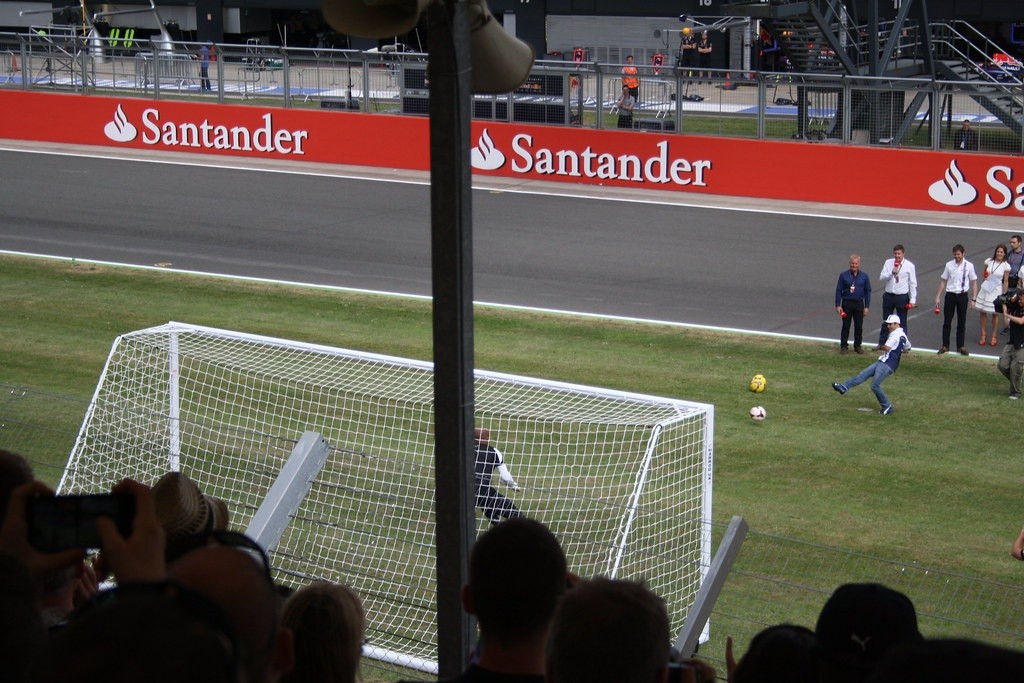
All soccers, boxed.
[749,406,767,421]
[749,373,767,393]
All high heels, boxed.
[990,336,997,346]
[980,334,987,345]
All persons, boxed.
[194,45,211,90]
[831,315,911,415]
[455,516,923,683]
[622,55,640,102]
[1000,235,1024,335]
[873,244,917,352]
[617,85,634,128]
[836,254,871,354]
[0,448,366,683]
[474,427,522,524]
[935,244,977,356]
[1011,529,1024,561]
[953,119,978,151]
[682,31,696,84]
[698,34,712,85]
[976,244,1011,346]
[997,288,1024,400]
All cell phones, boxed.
[32,493,137,549]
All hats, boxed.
[148,470,229,546]
[884,314,900,324]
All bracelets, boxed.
[972,300,976,302]
[892,271,895,274]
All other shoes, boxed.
[938,346,949,354]
[957,346,969,355]
[840,347,849,355]
[878,408,892,415]
[1001,327,1009,335]
[831,382,846,395]
[854,346,863,354]
[1008,395,1021,400]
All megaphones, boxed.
[322,0,437,40]
[467,0,535,95]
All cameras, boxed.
[667,663,702,683]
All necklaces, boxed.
[991,261,1002,274]
